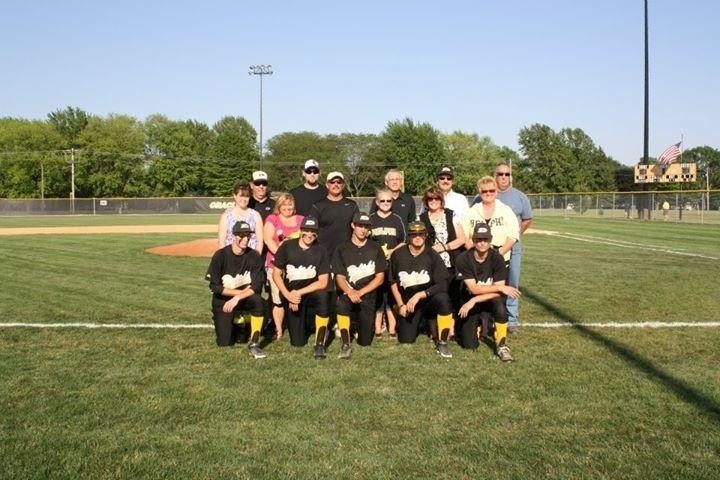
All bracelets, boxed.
[446,242,451,252]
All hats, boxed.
[437,167,453,176]
[232,221,255,234]
[473,223,491,239]
[327,171,344,182]
[300,216,319,230]
[305,159,319,170]
[408,221,426,234]
[353,212,372,226]
[251,171,268,182]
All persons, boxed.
[471,162,533,341]
[661,200,671,222]
[454,221,520,363]
[417,186,467,340]
[328,210,387,360]
[455,174,522,287]
[287,158,328,215]
[420,165,470,231]
[369,167,417,229]
[367,189,406,344]
[271,215,332,361]
[204,221,267,362]
[247,171,274,224]
[307,171,362,340]
[217,179,264,258]
[386,220,455,359]
[262,191,306,339]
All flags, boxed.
[657,140,683,168]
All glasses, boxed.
[330,178,342,184]
[380,199,392,203]
[305,169,319,174]
[481,189,496,193]
[427,196,440,202]
[497,172,510,176]
[439,176,452,181]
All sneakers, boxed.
[314,345,327,358]
[234,324,246,343]
[496,344,512,361]
[436,341,452,357]
[248,342,267,359]
[338,346,353,358]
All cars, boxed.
[600,197,696,210]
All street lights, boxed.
[248,64,274,171]
